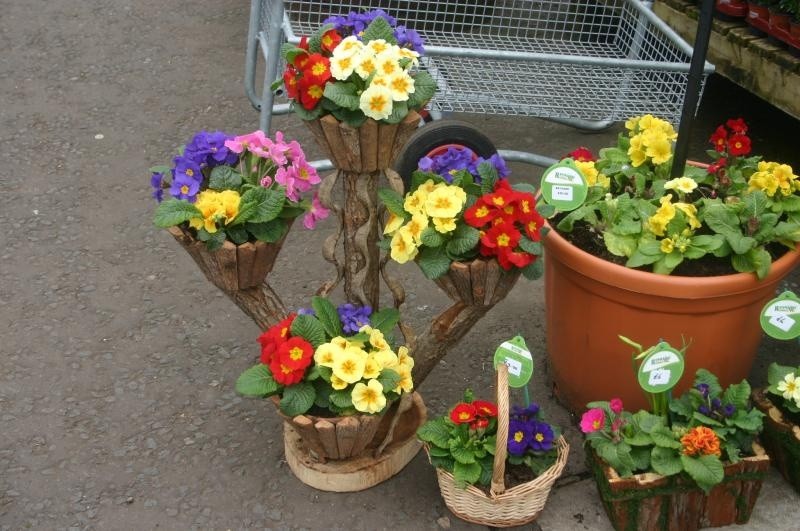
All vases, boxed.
[168,222,296,293]
[752,385,800,496]
[417,256,524,307]
[301,110,424,173]
[531,157,800,419]
[269,390,406,463]
[585,445,770,531]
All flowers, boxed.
[575,327,760,499]
[150,128,333,254]
[376,142,559,284]
[763,360,800,421]
[270,8,442,128]
[537,113,800,281]
[416,392,559,492]
[233,304,418,422]
